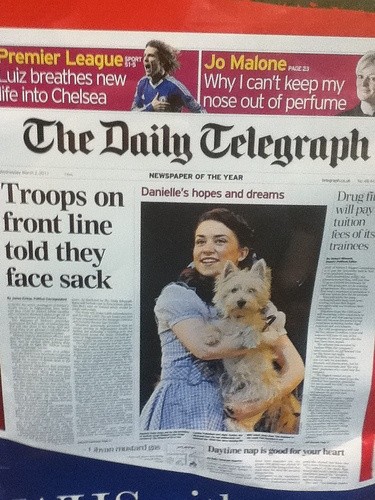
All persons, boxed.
[333,49,375,117]
[129,39,208,114]
[140,206,304,436]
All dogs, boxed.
[199,258,297,433]
